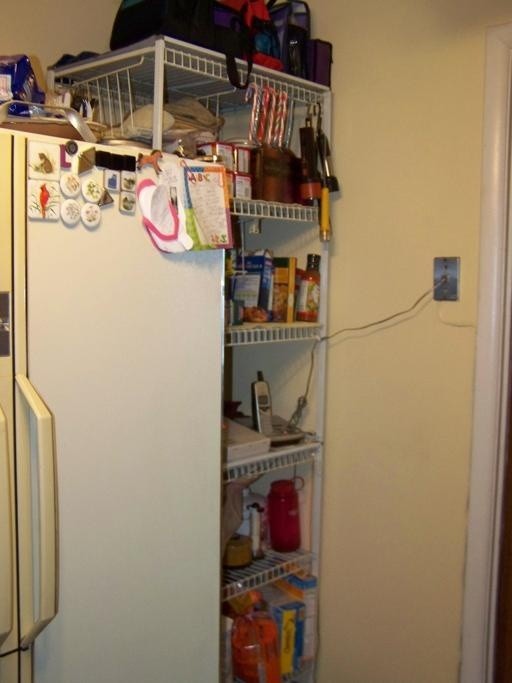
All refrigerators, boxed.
[0,125,226,683]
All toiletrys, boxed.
[249,503,264,559]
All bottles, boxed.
[295,254,323,321]
[268,473,306,553]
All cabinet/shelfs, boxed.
[40,36,331,683]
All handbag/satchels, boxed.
[109,0,283,90]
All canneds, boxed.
[238,147,256,170]
[203,140,234,173]
[225,175,257,198]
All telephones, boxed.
[236,371,305,447]
